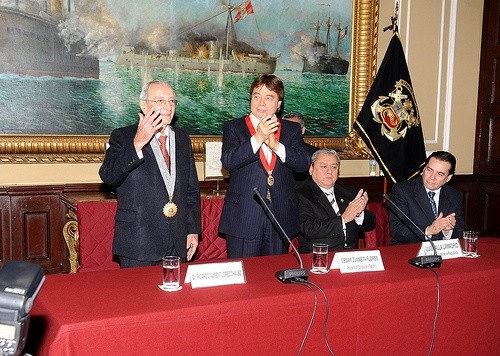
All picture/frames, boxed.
[0,0,380,164]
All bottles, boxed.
[369,151,376,176]
[379,166,384,176]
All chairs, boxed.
[63,194,395,273]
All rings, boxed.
[151,124,154,128]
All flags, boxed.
[352,27,427,186]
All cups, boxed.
[463,231,478,255]
[312,243,328,273]
[162,256,179,289]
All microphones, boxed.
[253,187,308,279]
[383,193,442,268]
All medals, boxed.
[162,201,178,218]
[267,175,275,186]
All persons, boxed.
[98,79,202,270]
[294,148,377,255]
[389,150,465,246]
[218,74,311,259]
[282,111,319,191]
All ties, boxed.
[324,190,340,216]
[158,135,171,173]
[429,191,437,218]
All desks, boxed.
[22,235,500,356]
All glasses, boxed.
[143,100,179,106]
